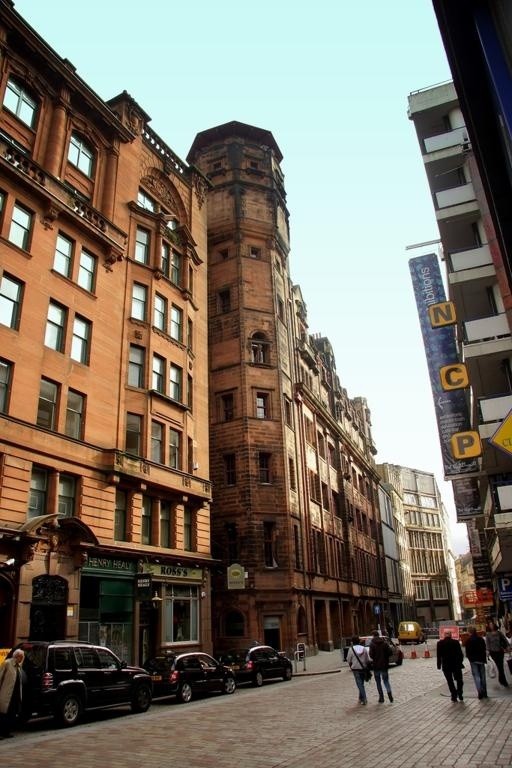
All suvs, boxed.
[4,633,154,727]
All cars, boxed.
[141,651,236,702]
[220,645,292,686]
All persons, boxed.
[370,630,393,703]
[387,622,393,638]
[466,625,488,699]
[347,635,369,704]
[486,621,511,686]
[501,624,506,636]
[495,618,501,632]
[437,630,465,702]
[0,648,25,740]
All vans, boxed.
[396,620,427,646]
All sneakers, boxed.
[477,689,488,699]
[359,697,367,705]
[450,690,463,701]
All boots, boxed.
[379,694,385,702]
[387,690,393,703]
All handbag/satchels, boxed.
[362,667,373,682]
[488,659,496,678]
